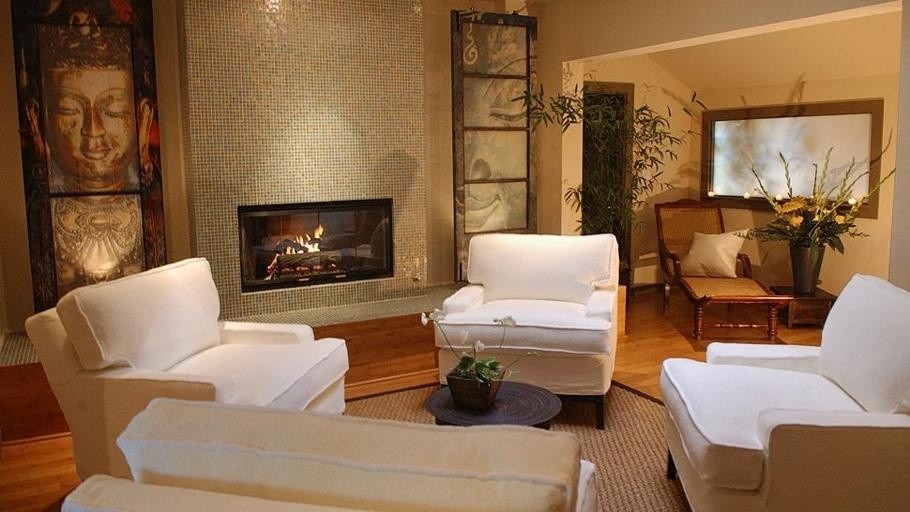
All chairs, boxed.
[660,273,909,512]
[436,232,621,429]
[795,198,893,341]
[57,397,597,511]
[654,200,794,343]
[24,255,350,480]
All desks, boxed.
[770,284,836,328]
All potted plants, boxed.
[517,66,709,337]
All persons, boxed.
[16,0,168,310]
[461,20,536,233]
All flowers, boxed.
[735,126,896,259]
[418,307,542,385]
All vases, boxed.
[447,372,507,414]
[790,248,826,295]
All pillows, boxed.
[680,228,748,279]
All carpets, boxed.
[327,375,689,512]
[0,331,40,369]
